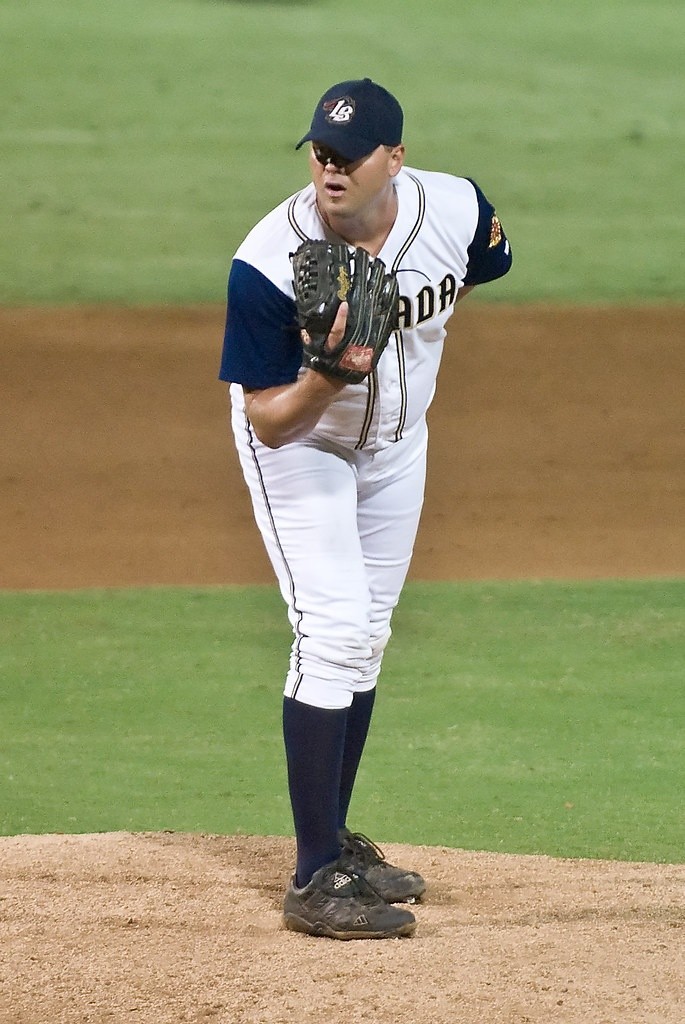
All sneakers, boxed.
[337,828,426,903]
[283,859,418,940]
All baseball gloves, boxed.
[288,240,402,386]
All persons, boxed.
[217,81,512,944]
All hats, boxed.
[295,78,403,162]
